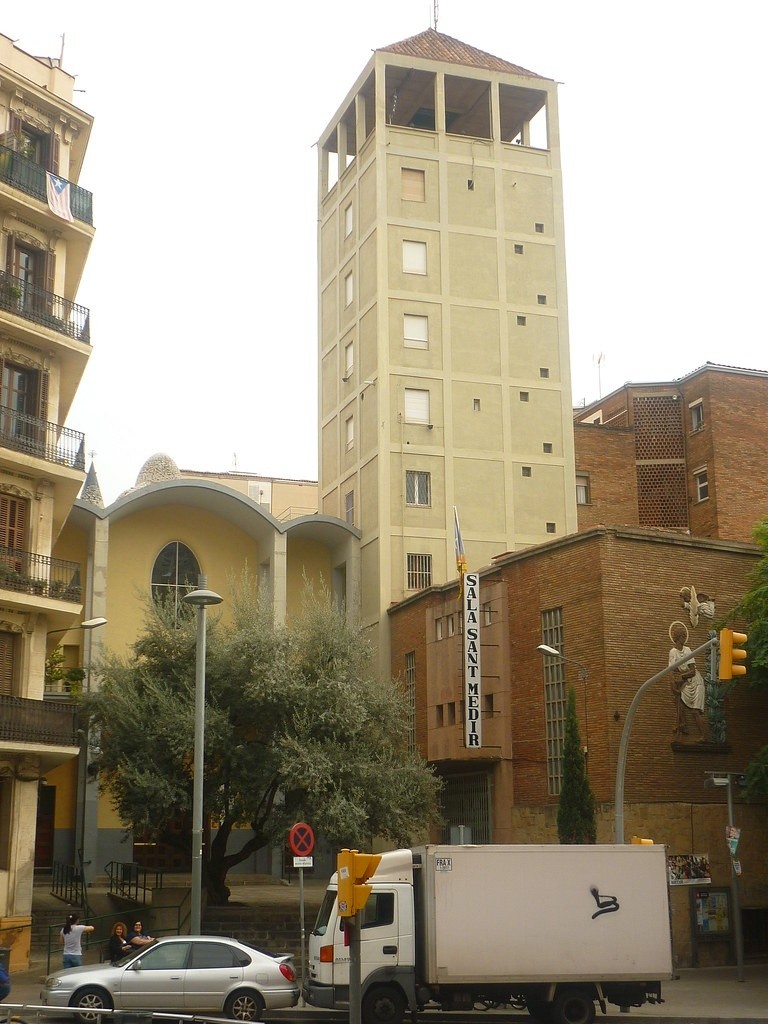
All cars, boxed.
[40,935,300,1022]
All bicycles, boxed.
[1,1016,27,1024]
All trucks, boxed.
[302,843,681,1024]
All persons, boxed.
[0,961,11,1001]
[670,855,710,879]
[124,921,155,950]
[58,914,94,969]
[108,922,134,967]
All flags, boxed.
[453,507,467,600]
[46,171,75,223]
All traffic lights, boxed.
[631,836,654,846]
[718,628,748,679]
[336,849,383,917]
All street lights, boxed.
[706,777,745,985]
[182,588,224,936]
